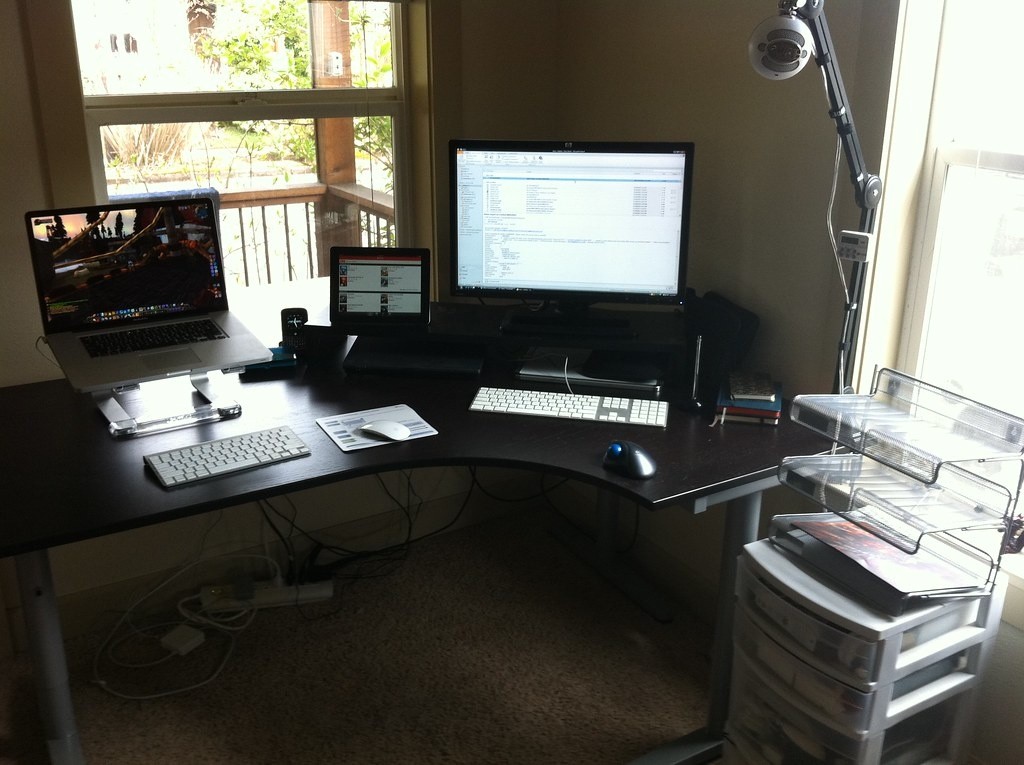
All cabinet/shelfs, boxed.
[720,367,1023,764]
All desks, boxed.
[2,299,853,765]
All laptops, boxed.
[23,198,272,396]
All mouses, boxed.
[359,419,411,441]
[601,438,657,483]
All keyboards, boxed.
[469,385,670,430]
[142,425,310,489]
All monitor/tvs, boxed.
[439,139,696,310]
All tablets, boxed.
[330,246,431,328]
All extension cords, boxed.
[197,583,335,609]
